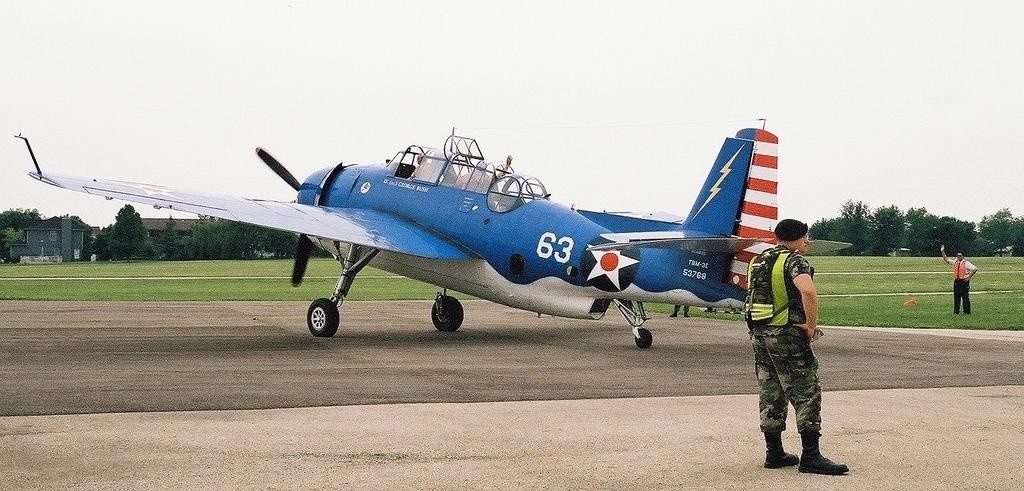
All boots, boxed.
[763,430,799,467]
[799,433,850,474]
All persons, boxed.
[725,307,742,314]
[744,219,849,475]
[940,244,977,314]
[408,153,432,181]
[463,155,513,193]
[670,305,691,317]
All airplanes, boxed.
[7,98,861,372]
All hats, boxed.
[776,219,809,241]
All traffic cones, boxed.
[902,297,918,307]
